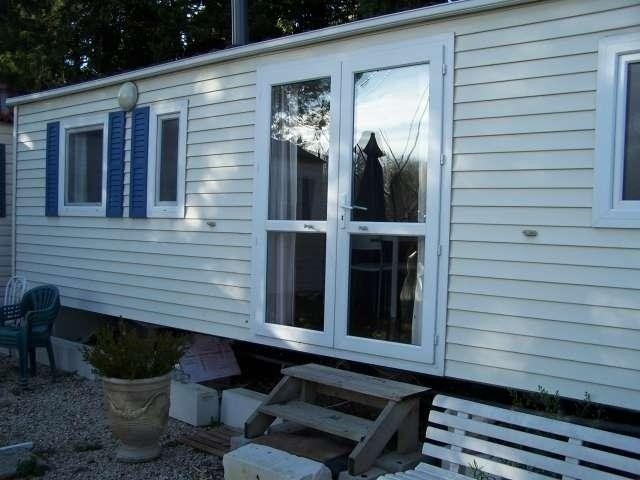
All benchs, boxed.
[375,395,640,480]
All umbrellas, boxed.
[354,133,387,222]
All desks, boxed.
[382,235,416,318]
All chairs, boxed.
[350,234,391,318]
[1,276,59,389]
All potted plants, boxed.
[78,316,195,462]
